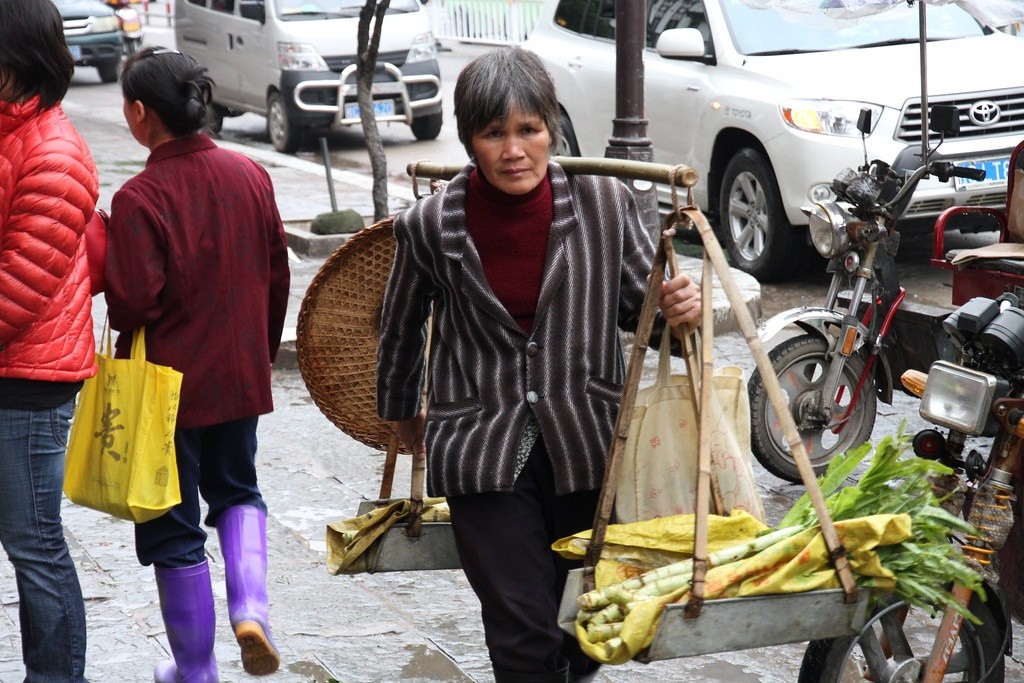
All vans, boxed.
[173,0,444,155]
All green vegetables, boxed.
[580,420,988,658]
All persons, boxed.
[376,45,703,683]
[1,0,98,683]
[104,46,291,683]
[999,140,1024,244]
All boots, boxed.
[217,504,280,676]
[153,557,220,683]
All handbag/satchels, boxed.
[616,318,766,527]
[60,306,183,522]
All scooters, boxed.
[105,0,146,58]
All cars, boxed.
[40,0,123,81]
[513,0,1024,278]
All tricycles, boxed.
[738,104,1024,490]
[780,285,1024,683]
